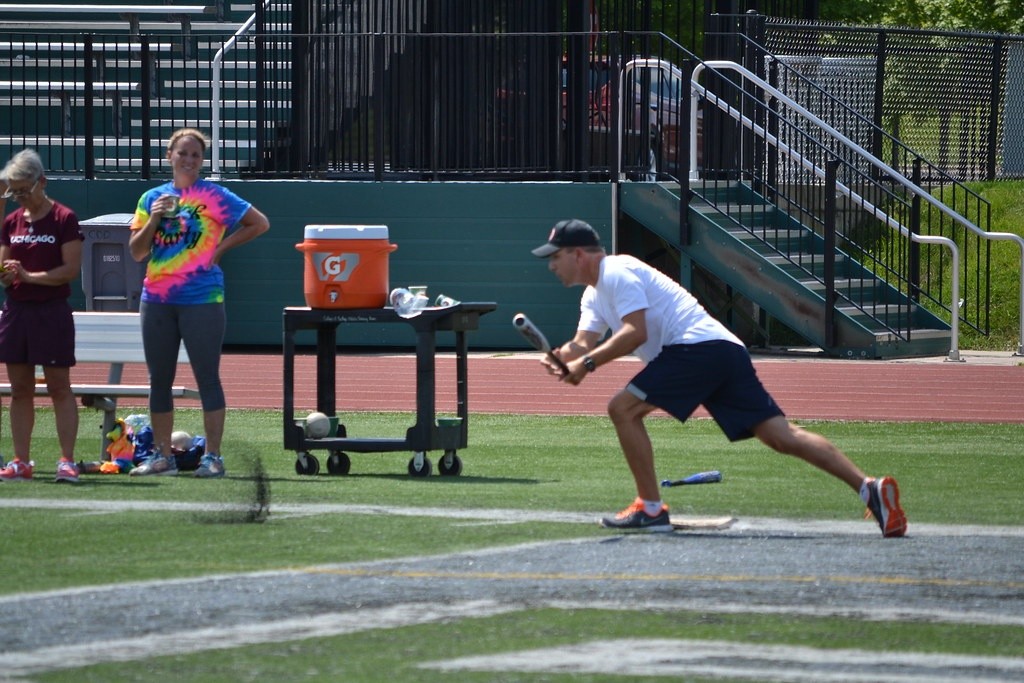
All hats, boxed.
[531,219,599,256]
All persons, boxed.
[129,130,269,479]
[0,149,85,484]
[530,220,906,538]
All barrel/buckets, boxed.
[295,224,398,310]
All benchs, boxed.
[0,310,201,462]
[0,82,142,138]
[0,42,184,100]
[0,4,219,62]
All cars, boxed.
[496,51,703,169]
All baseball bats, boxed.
[661,470,723,487]
[513,314,569,375]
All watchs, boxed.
[584,354,595,372]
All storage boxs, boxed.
[294,225,398,309]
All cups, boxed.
[408,286,428,298]
[161,194,180,219]
[435,294,461,308]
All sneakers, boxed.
[194,454,225,477]
[56,457,79,481]
[0,458,33,481]
[129,447,178,476]
[864,476,907,537]
[599,497,674,532]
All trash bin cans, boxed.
[77,212,147,313]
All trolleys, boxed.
[283,301,498,477]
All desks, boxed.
[280,301,498,477]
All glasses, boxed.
[1,180,40,202]
